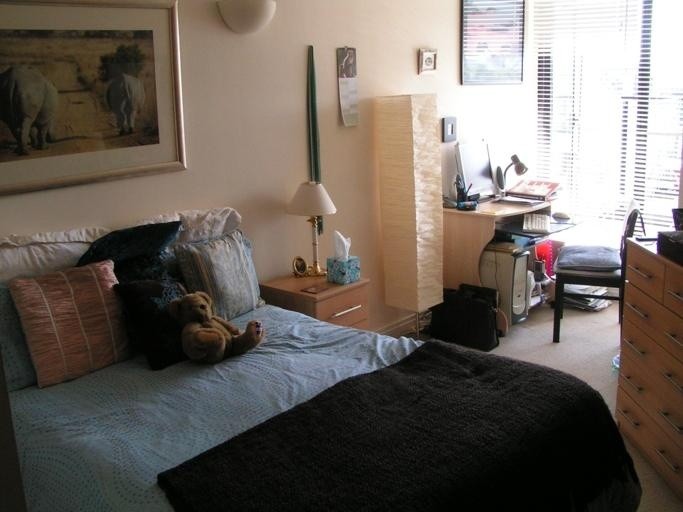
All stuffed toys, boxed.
[163,291,265,364]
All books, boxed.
[491,195,544,206]
[505,177,561,202]
[563,282,612,312]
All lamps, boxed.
[286,181,337,278]
[495,153,529,189]
[217,1,276,34]
[375,94,444,339]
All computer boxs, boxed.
[478,249,530,325]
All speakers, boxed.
[495,166,505,197]
[453,173,461,200]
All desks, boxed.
[443,188,584,337]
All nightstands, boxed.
[259,275,371,330]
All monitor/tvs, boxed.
[454,139,497,203]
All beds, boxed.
[0,303,642,512]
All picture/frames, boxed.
[442,117,457,142]
[0,0,187,196]
[417,48,437,75]
[460,0,526,86]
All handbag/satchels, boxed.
[456,284,498,309]
[429,287,498,351]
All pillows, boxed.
[555,245,622,272]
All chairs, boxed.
[552,195,638,342]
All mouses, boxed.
[551,212,569,218]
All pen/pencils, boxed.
[467,184,472,193]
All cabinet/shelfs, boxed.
[614,237,683,504]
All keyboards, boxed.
[522,213,550,234]
